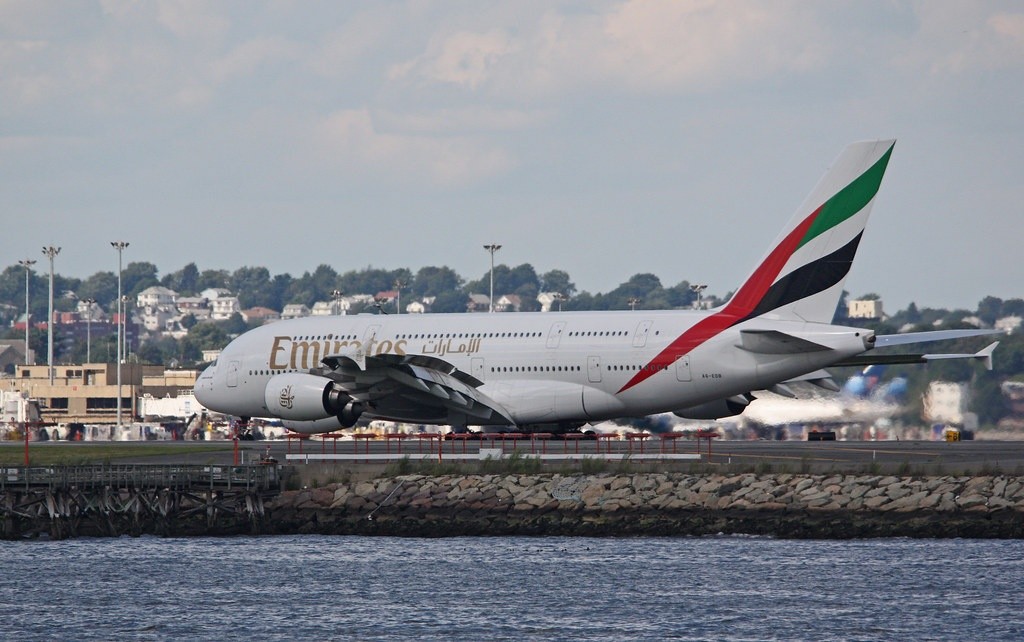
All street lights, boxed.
[388,281,407,314]
[689,284,707,312]
[19,258,38,366]
[108,240,130,429]
[81,297,97,363]
[328,289,343,316]
[482,244,501,313]
[41,244,63,386]
[552,291,569,312]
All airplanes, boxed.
[194,136,1004,447]
[575,355,914,438]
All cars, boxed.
[370,420,414,438]
[41,422,77,443]
[252,416,301,442]
